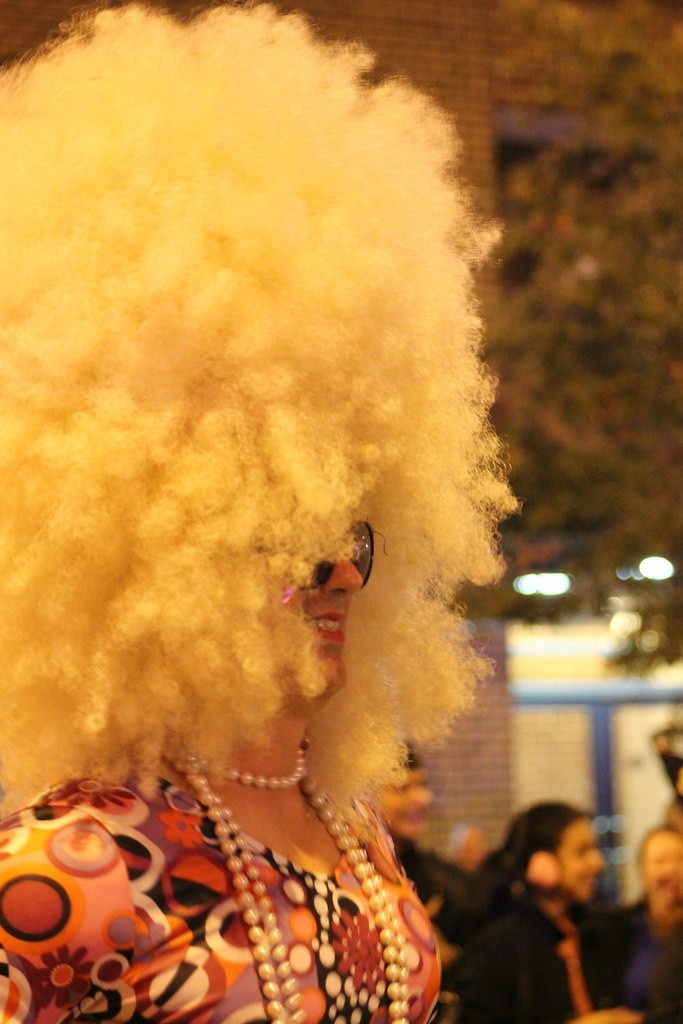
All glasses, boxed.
[298,522,374,591]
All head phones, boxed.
[525,809,575,891]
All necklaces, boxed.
[158,743,410,1024]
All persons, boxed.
[0,0,522,1024]
[368,753,683,1024]
